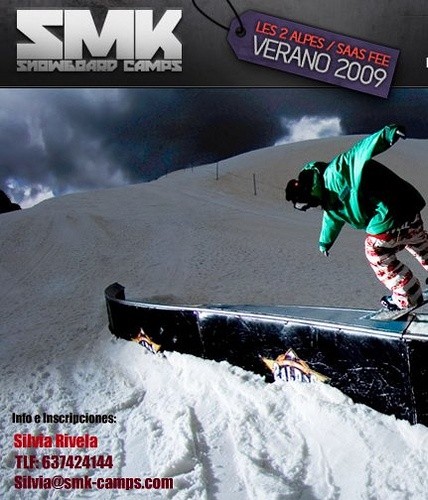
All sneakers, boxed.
[380,295,400,311]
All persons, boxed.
[285,121,427,321]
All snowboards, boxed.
[370,290,428,321]
